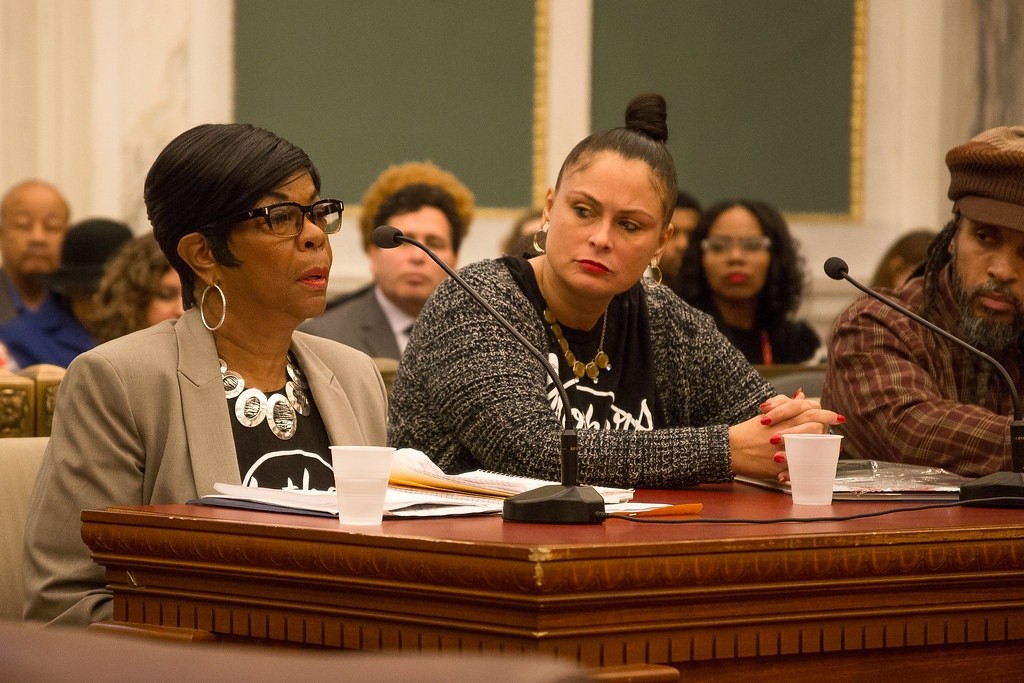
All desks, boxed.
[79,478,1024,683]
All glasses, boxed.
[238,197,344,238]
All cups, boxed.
[781,434,845,506]
[328,445,397,526]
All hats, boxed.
[59,219,135,279]
[946,126,1024,230]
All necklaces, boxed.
[543,306,609,378]
[220,356,310,440]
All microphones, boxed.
[825,257,1024,510]
[372,226,605,524]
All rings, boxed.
[824,423,833,434]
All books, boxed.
[388,469,635,507]
[735,475,960,500]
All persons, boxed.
[821,126,1023,478]
[675,198,821,365]
[869,230,938,284]
[388,93,845,488]
[502,211,544,257]
[295,159,476,361]
[0,178,71,324]
[644,191,702,291]
[21,123,388,625]
[0,218,184,373]
